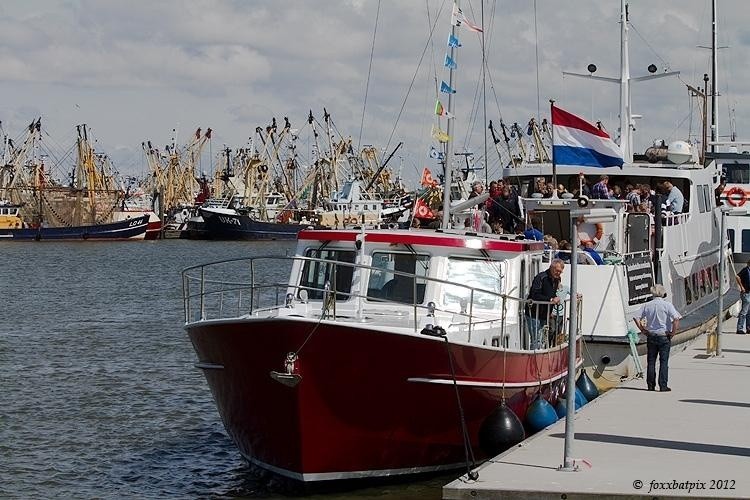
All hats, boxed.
[650,283,665,295]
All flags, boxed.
[551,106,626,170]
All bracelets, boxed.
[550,299,553,301]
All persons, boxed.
[735,259,750,335]
[549,275,583,348]
[466,175,685,266]
[633,284,682,392]
[524,258,565,350]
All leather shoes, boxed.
[660,387,670,391]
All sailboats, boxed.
[684,0,750,293]
[178,1,587,485]
[0,109,481,240]
[498,0,733,375]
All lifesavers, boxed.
[727,187,747,206]
[575,215,604,247]
